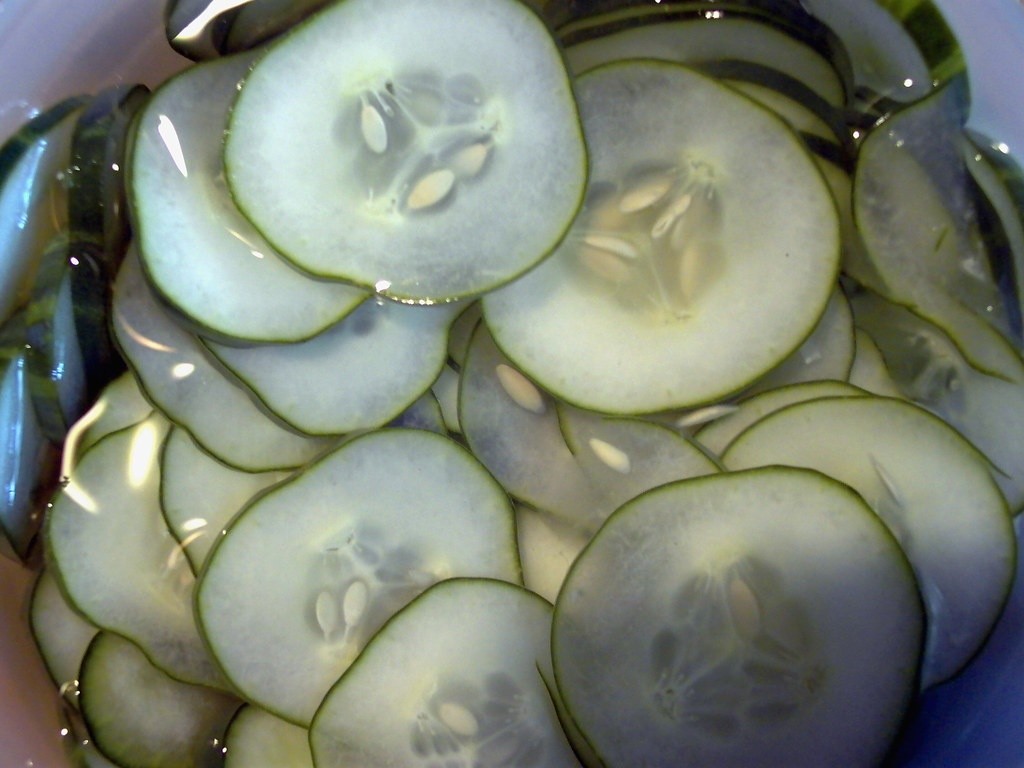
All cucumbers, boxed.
[0,0,1024,767]
